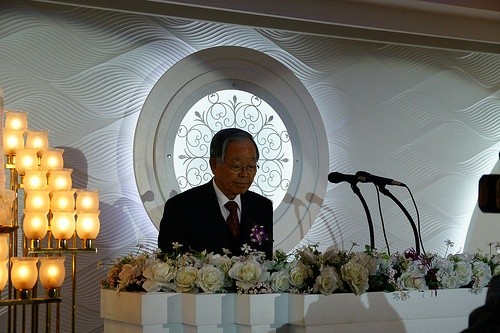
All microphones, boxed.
[328,172,369,184]
[356,171,406,187]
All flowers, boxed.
[94,242,500,301]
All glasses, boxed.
[223,161,260,173]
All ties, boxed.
[224,201,240,239]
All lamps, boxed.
[1,110,102,299]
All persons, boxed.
[158,128,273,261]
[461,274,500,333]
